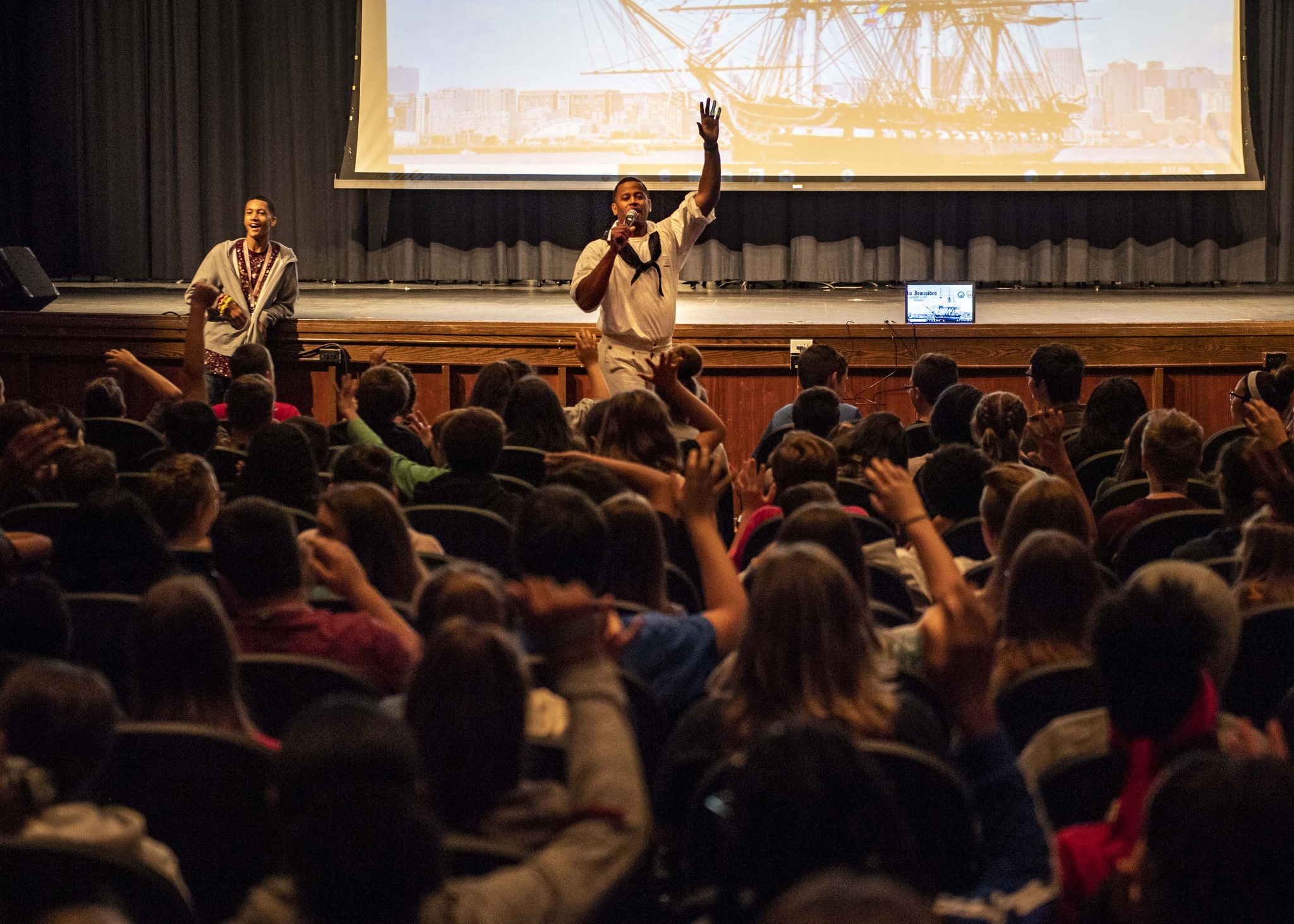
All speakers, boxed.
[0,247,61,311]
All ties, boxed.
[599,228,664,297]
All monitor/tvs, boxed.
[905,281,976,324]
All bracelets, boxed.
[217,294,235,318]
[902,514,929,528]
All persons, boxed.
[184,195,300,407]
[570,97,721,398]
[0,328,1294,924]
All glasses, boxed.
[902,384,914,391]
[1025,370,1038,379]
[1229,390,1247,402]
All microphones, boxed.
[623,209,637,227]
